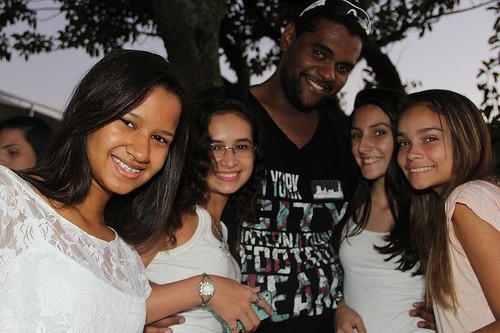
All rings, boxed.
[254,295,264,304]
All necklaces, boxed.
[211,215,240,285]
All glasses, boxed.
[299,0,371,36]
[211,143,257,158]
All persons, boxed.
[133,91,274,333]
[382,89,500,333]
[145,0,437,332]
[0,115,53,171]
[1,49,190,333]
[326,88,436,333]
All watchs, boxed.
[198,273,216,305]
